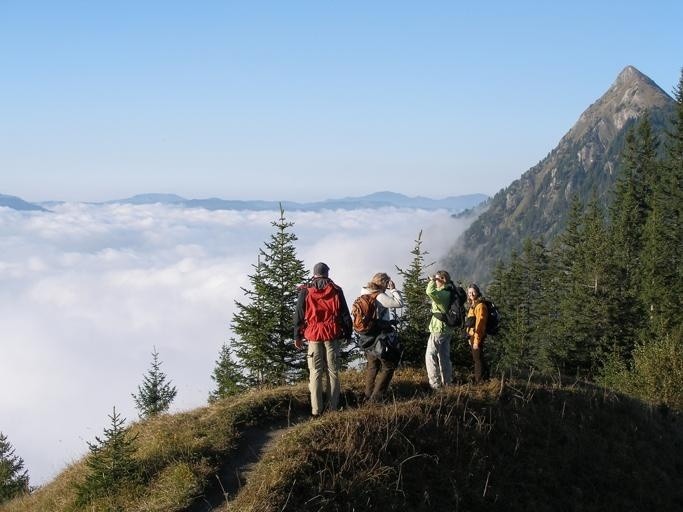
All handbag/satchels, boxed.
[335,288,353,341]
[374,336,402,363]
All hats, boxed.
[314,262,329,274]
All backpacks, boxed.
[473,300,500,336]
[430,287,467,327]
[351,291,383,333]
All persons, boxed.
[359,270,403,407]
[294,262,352,421]
[424,270,455,391]
[465,283,488,385]
